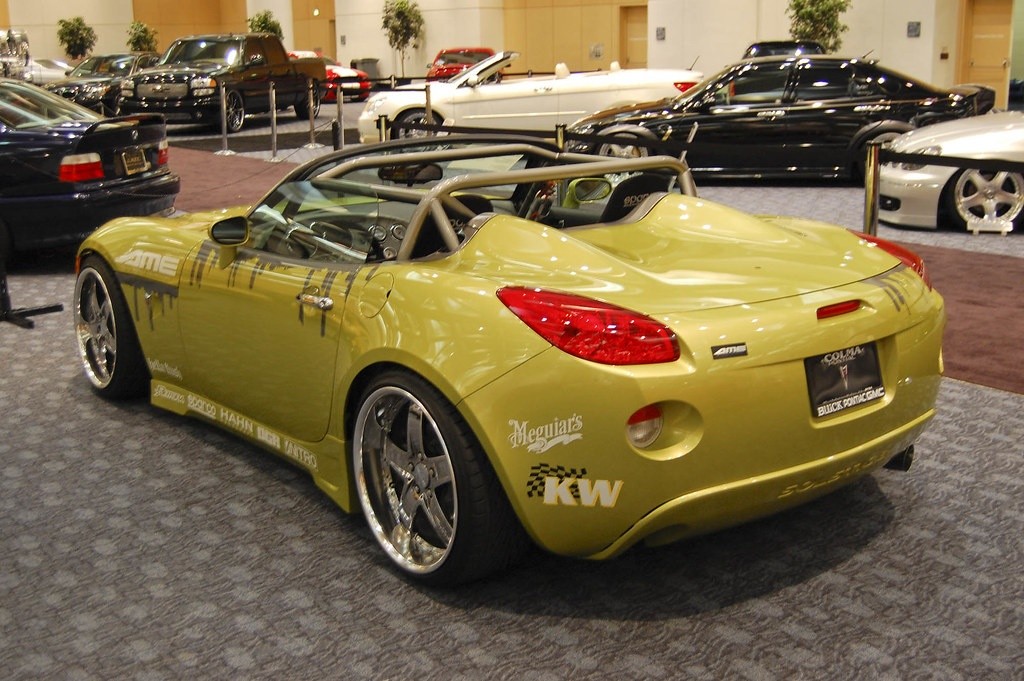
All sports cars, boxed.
[73,133,944,588]
[358,51,704,152]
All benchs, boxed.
[609,61,621,73]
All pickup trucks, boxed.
[117,32,327,131]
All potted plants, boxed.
[381,0,426,84]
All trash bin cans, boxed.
[350,58,380,92]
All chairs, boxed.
[600,175,667,224]
[555,61,571,79]
[409,194,493,259]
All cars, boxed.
[877,110,1024,234]
[426,48,511,86]
[0,75,181,267]
[566,40,994,185]
[285,51,372,103]
[28,51,161,115]
